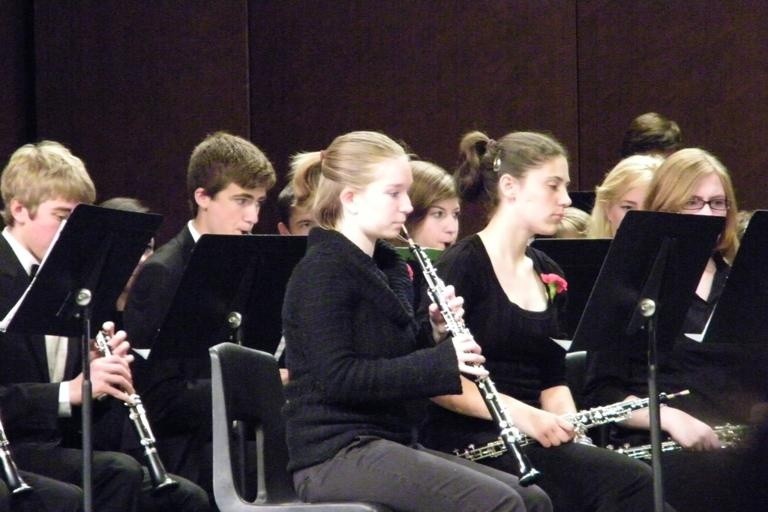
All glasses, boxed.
[683,195,734,214]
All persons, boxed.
[417,131,726,512]
[99,197,156,313]
[280,131,554,510]
[585,147,766,509]
[0,468,86,512]
[105,131,287,509]
[0,140,212,512]
[275,112,683,249]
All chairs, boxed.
[208,341,385,511]
[562,349,586,383]
